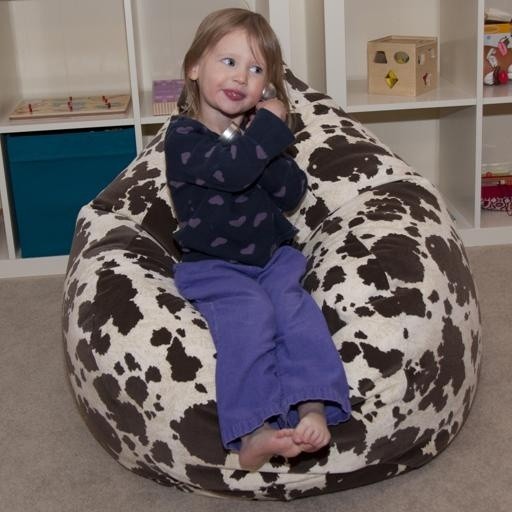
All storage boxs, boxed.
[4,127,135,258]
[483,22,512,86]
[481,174,512,213]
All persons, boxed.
[165,6,353,471]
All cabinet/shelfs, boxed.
[1,0,512,282]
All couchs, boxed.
[61,54,483,503]
